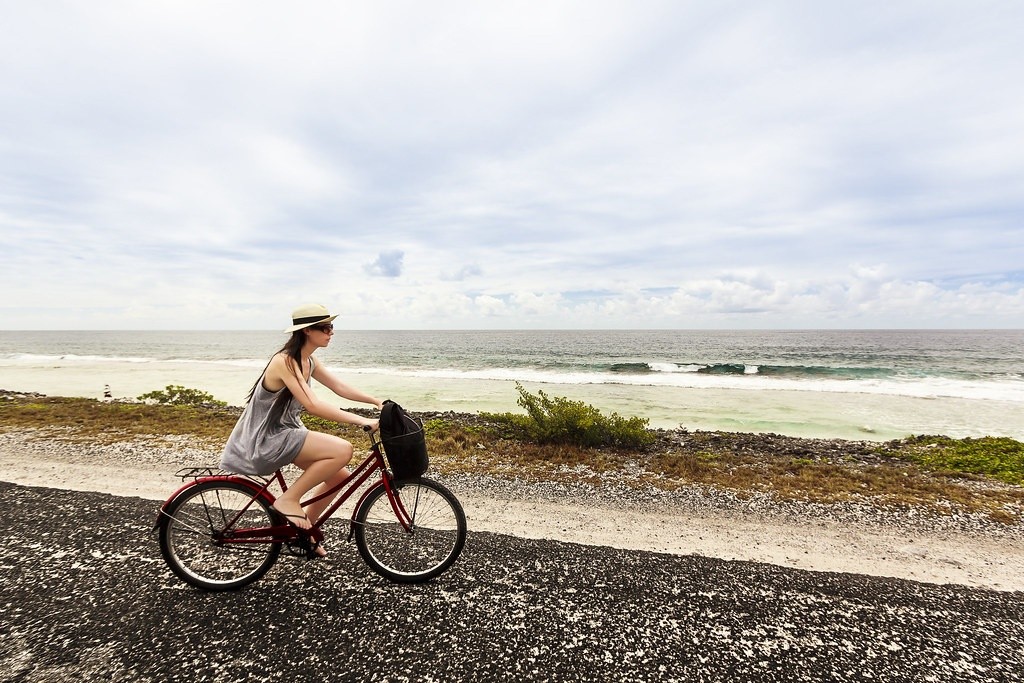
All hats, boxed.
[284,303,339,333]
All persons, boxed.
[219,305,382,557]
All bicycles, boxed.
[150,409,467,593]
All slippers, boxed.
[268,504,313,532]
[310,542,326,558]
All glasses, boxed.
[310,323,333,334]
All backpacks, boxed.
[379,399,428,480]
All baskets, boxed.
[382,417,428,480]
